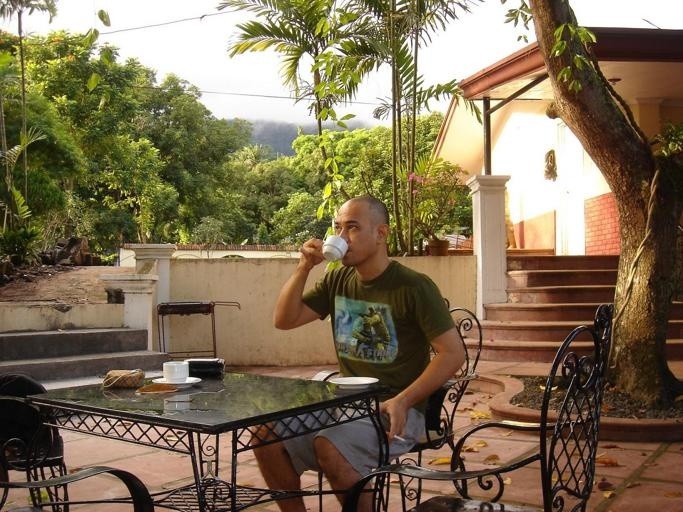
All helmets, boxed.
[328,376,380,390]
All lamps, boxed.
[295,296,482,512]
[0,396,153,511]
[0,373,69,511]
[343,302,613,512]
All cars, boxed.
[152,377,202,388]
[162,361,189,382]
[322,234,348,259]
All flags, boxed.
[426,238,449,257]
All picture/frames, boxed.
[28,371,390,512]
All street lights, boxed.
[103,368,144,389]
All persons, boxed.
[250,195,468,511]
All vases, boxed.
[397,168,466,243]
